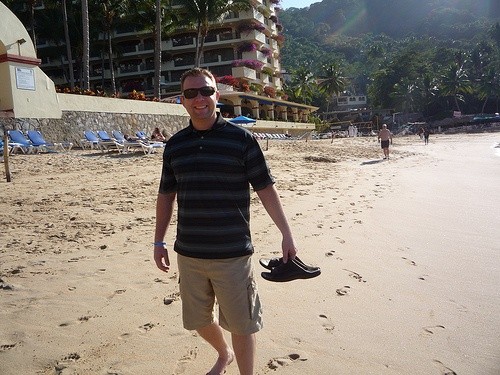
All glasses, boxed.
[182,86,217,99]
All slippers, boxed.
[261,260,321,282]
[259,255,320,271]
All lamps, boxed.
[18,39,26,44]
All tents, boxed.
[229,114,256,135]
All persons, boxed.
[154,68,298,375]
[424,128,429,145]
[378,123,392,159]
[419,128,423,139]
[124,134,149,145]
[151,127,166,142]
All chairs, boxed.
[80,127,165,154]
[0,128,74,155]
[252,133,294,140]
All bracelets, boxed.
[154,243,166,246]
